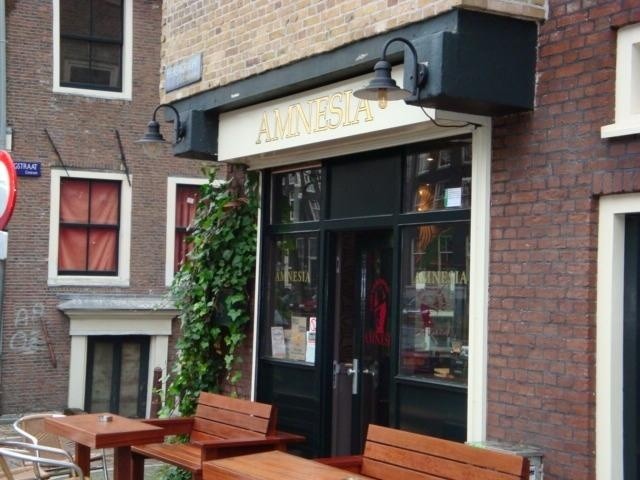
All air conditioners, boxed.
[465,438,543,480]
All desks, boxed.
[203,451,372,480]
[46,412,165,480]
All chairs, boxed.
[0,441,90,480]
[13,413,109,480]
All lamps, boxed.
[353,36,428,110]
[133,103,184,158]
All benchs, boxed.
[131,392,306,480]
[313,423,528,480]
